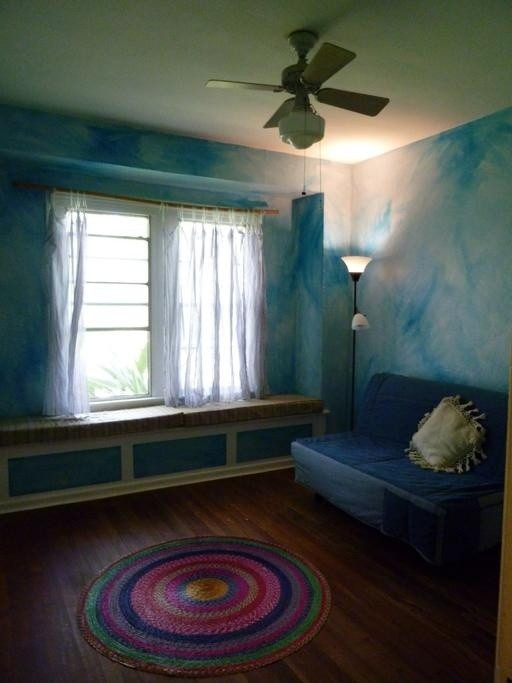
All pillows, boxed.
[404,394,488,474]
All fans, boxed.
[204,31,390,129]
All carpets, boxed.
[76,536,333,679]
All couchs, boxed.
[290,373,507,568]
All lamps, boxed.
[278,86,325,151]
[341,255,374,431]
